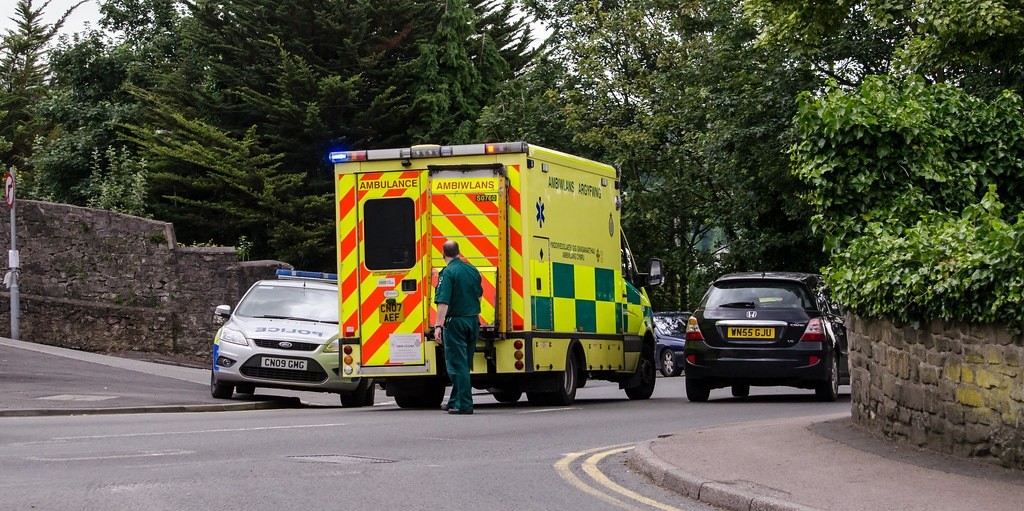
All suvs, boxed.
[683,272,851,404]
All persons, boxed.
[434,240,482,414]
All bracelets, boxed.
[435,325,441,328]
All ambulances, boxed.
[329,140,666,407]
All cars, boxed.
[210,270,376,408]
[655,311,696,377]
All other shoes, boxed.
[449,407,473,414]
[441,404,453,411]
